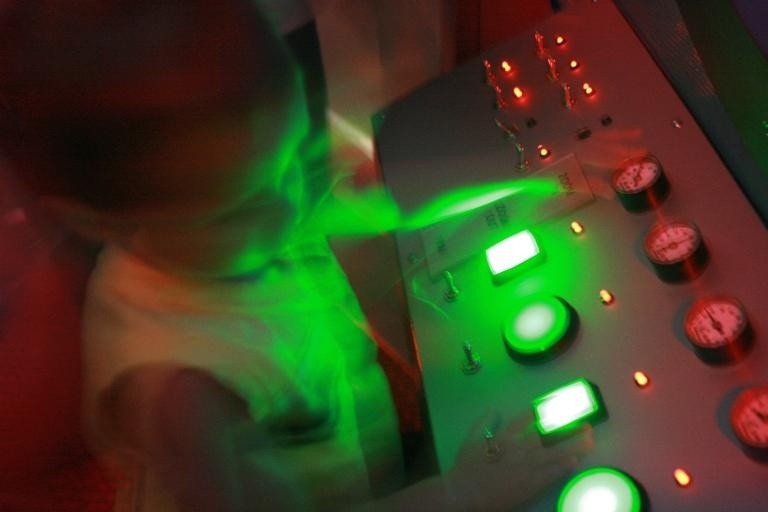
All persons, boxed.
[0,0,595,512]
